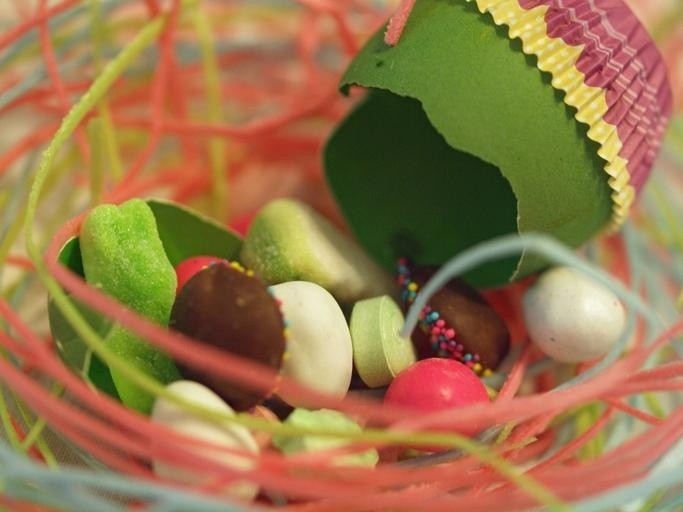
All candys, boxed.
[152,198,626,512]
